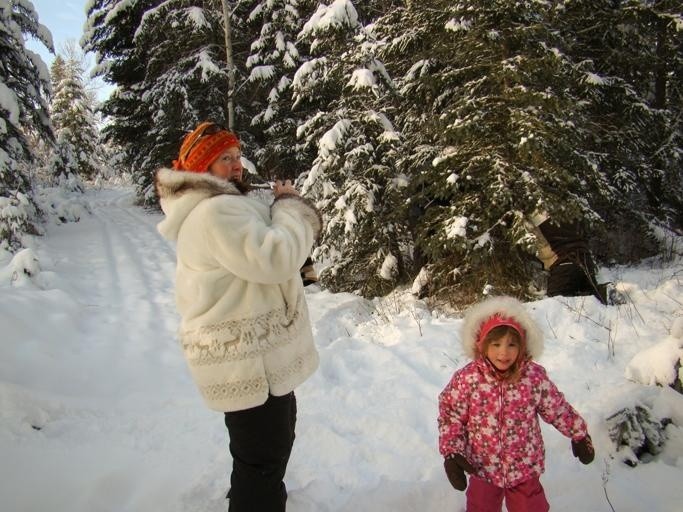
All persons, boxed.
[438,296,595,512]
[157,118,322,510]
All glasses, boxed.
[182,122,232,162]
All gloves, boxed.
[571,436,597,464]
[444,453,476,491]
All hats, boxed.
[172,121,240,174]
[479,315,526,352]
